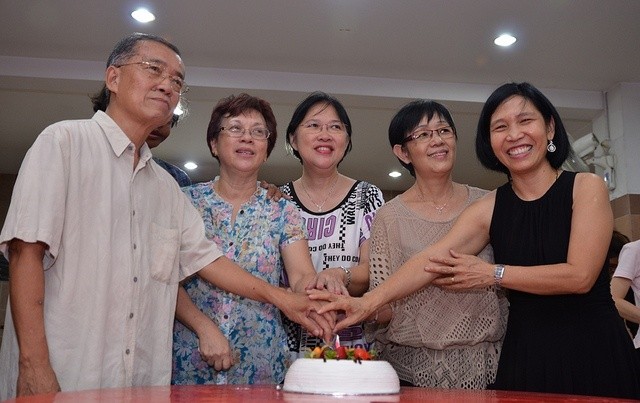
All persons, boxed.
[604,230,639,338]
[306,81,639,398]
[86,83,191,188]
[170,91,338,387]
[610,237,639,351]
[0,32,338,399]
[260,91,386,359]
[360,98,512,392]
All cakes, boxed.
[283,334,401,395]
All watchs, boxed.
[494,263,507,291]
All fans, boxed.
[557,127,617,192]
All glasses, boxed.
[396,125,457,142]
[293,120,347,134]
[113,60,191,96]
[214,124,271,141]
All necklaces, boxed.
[555,168,559,181]
[414,181,455,214]
[300,171,339,214]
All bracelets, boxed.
[338,266,352,289]
[366,308,379,326]
